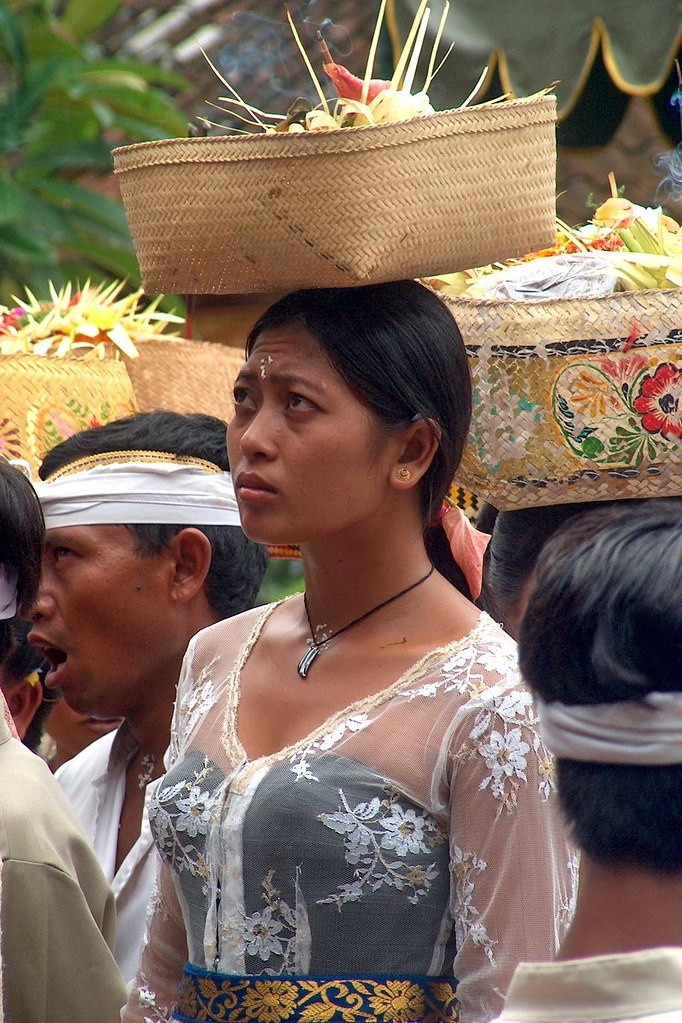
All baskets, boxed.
[0,93,682,561]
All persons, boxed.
[0,410,271,1023]
[119,279,579,1023]
[477,499,682,1023]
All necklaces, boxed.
[298,565,434,681]
[131,743,160,791]
